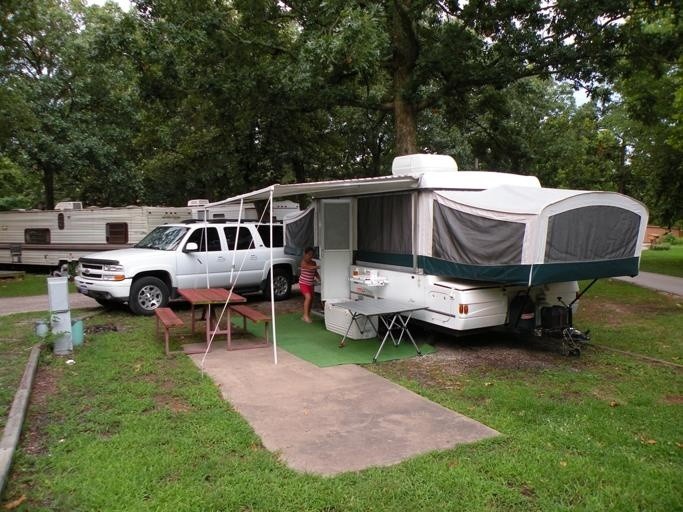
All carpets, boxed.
[231,311,437,367]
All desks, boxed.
[176,287,246,353]
[330,299,428,363]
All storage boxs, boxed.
[324,302,379,340]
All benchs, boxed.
[153,304,272,354]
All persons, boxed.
[297,247,320,324]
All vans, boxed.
[74,223,301,316]
